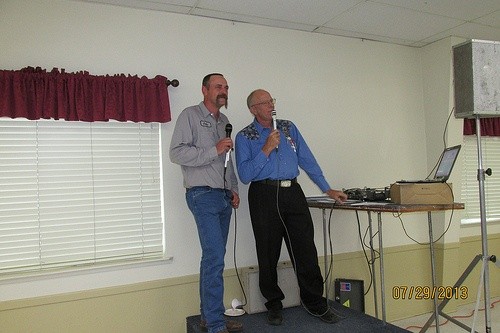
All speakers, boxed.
[453,39,500,119]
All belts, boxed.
[252,179,296,187]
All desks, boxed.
[306,196,466,333]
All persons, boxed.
[233,90,348,324]
[169,73,244,333]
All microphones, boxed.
[271,110,279,149]
[225,124,232,138]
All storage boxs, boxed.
[391,182,455,205]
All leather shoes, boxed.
[200,319,244,331]
[267,308,284,325]
[309,305,339,323]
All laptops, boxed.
[397,144,461,183]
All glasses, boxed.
[250,98,276,106]
